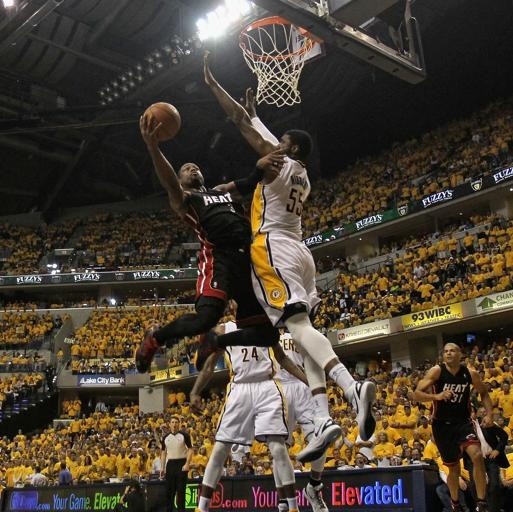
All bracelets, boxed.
[124,493,127,496]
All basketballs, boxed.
[142,101,180,141]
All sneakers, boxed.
[475,501,490,512]
[293,418,343,462]
[133,325,165,372]
[449,499,464,512]
[301,481,330,512]
[351,380,377,442]
[277,500,289,512]
[192,326,218,372]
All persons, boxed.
[135,112,288,373]
[202,49,377,460]
[159,416,193,510]
[1,201,193,278]
[189,323,329,512]
[222,338,513,511]
[309,236,513,337]
[302,99,513,240]
[113,479,147,511]
[1,288,236,376]
[0,384,226,491]
[0,371,42,407]
